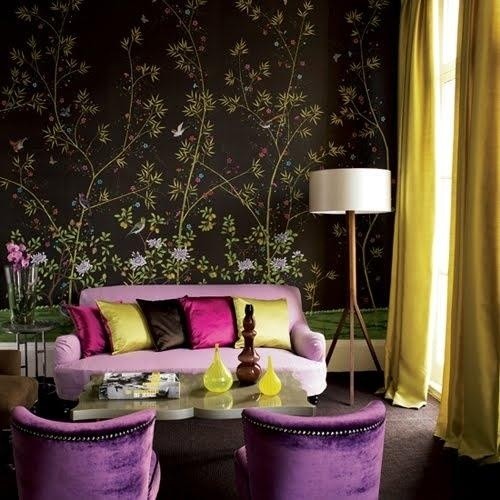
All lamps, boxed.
[309,167,392,403]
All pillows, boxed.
[94,299,154,357]
[62,302,105,359]
[231,296,291,352]
[176,296,238,350]
[136,295,195,350]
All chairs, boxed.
[0,405,161,500]
[0,349,39,429]
[224,400,390,500]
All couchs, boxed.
[57,284,328,403]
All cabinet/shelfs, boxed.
[7,326,48,378]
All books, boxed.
[97,371,181,401]
